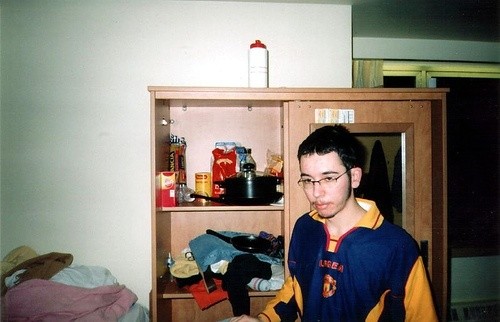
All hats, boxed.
[0,245,74,301]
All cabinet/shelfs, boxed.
[147,85,451,322]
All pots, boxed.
[207,229,271,252]
[190,176,279,204]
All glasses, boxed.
[298,171,350,189]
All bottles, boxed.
[240,149,255,178]
